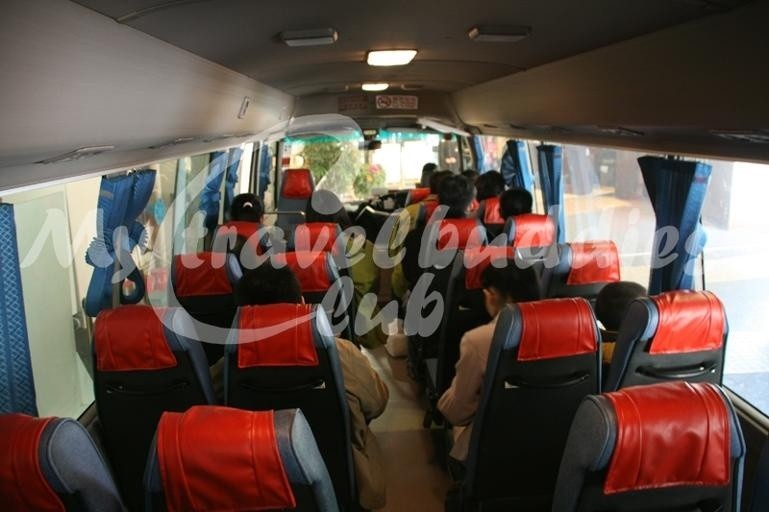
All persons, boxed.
[207,263,390,511]
[305,189,380,300]
[205,192,273,249]
[384,162,542,458]
[592,280,649,389]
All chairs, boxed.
[0,164,748,512]
